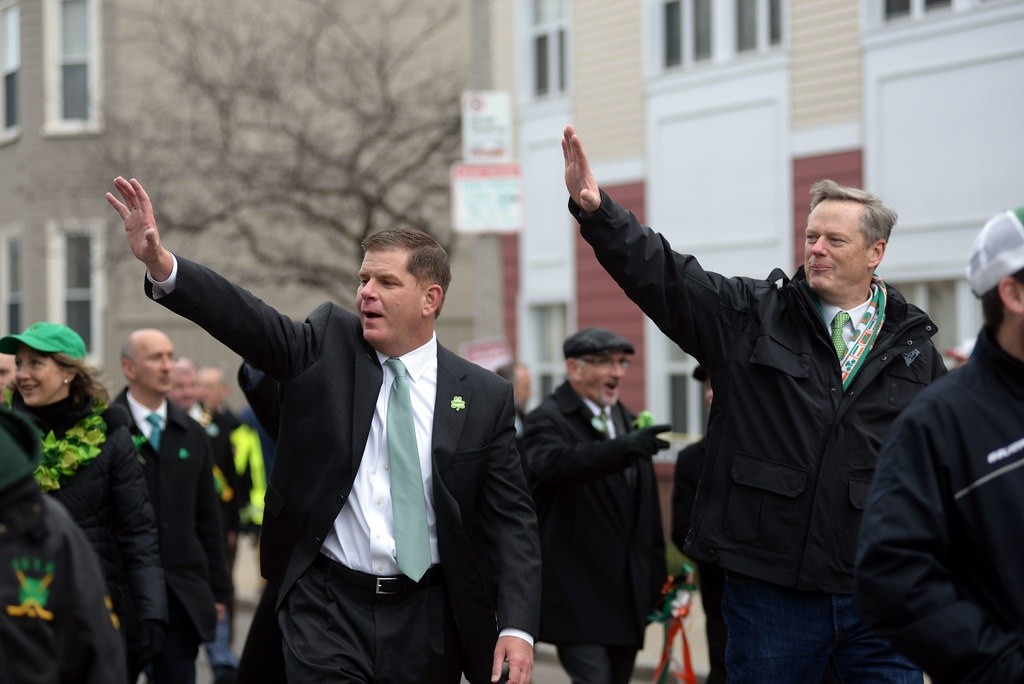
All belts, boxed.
[313,553,434,596]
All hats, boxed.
[965,207,1024,295]
[562,328,637,359]
[0,321,86,364]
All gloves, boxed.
[620,423,672,461]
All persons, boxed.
[0,206,1024,684]
[106,175,546,684]
[562,122,950,684]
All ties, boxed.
[147,413,164,452]
[832,311,850,358]
[387,357,433,584]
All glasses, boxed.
[577,353,632,370]
[130,353,179,364]
